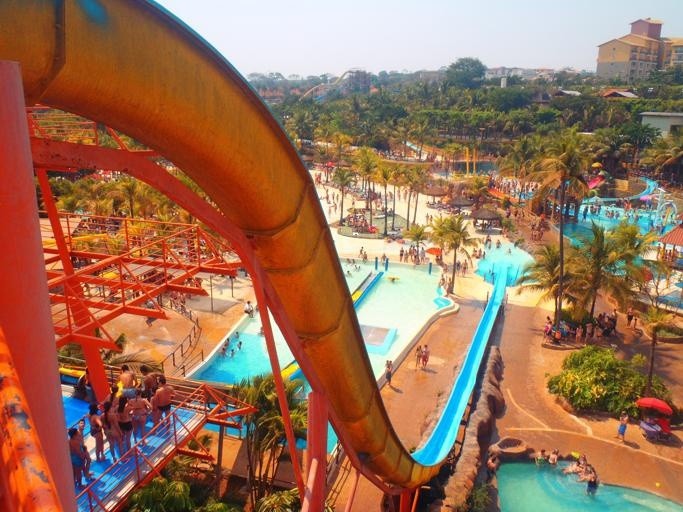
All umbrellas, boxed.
[636,395,674,417]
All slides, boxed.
[0,0,504,487]
[205,271,385,402]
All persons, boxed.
[624,307,635,329]
[66,362,178,491]
[413,343,429,370]
[219,330,243,359]
[613,410,629,441]
[540,307,618,345]
[382,358,393,386]
[561,452,599,497]
[547,447,559,466]
[168,277,201,313]
[484,453,498,485]
[242,299,254,319]
[141,299,158,328]
[312,169,678,297]
[533,447,547,468]
